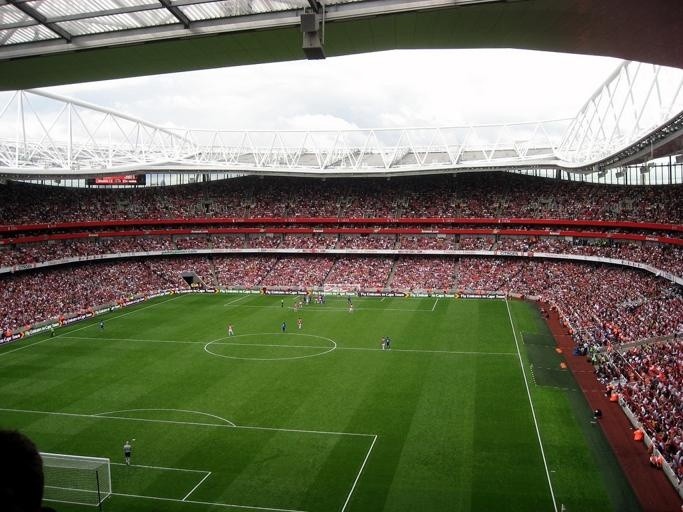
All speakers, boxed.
[301,13,327,60]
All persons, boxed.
[226,322,234,338]
[296,317,302,329]
[0,426,44,511]
[191,172,540,315]
[280,320,287,334]
[380,336,386,350]
[123,439,132,466]
[385,337,391,349]
[0,179,191,344]
[540,180,683,489]
